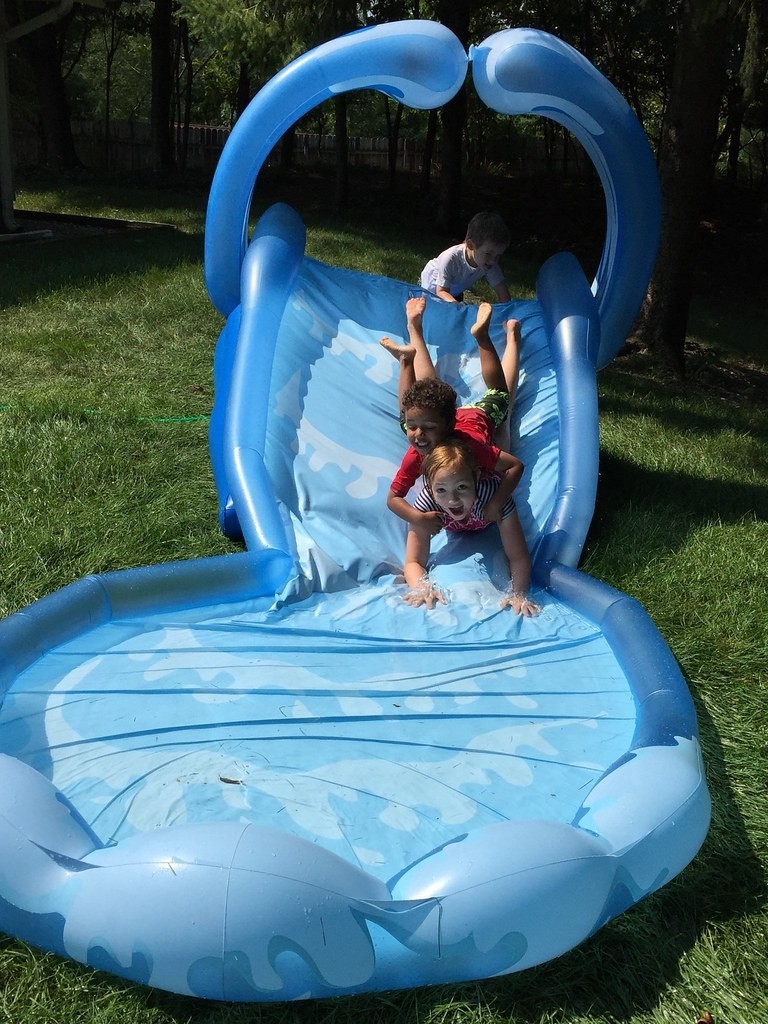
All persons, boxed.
[379,298,537,617]
[418,210,515,304]
